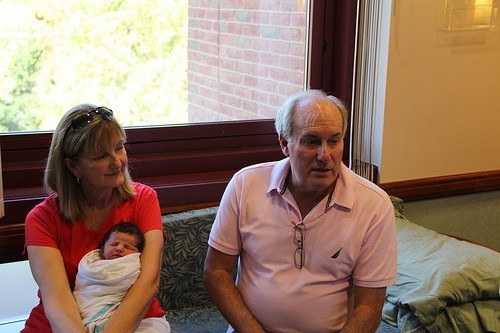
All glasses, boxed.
[291,221,306,269]
[63,106,113,134]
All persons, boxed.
[73,222,144,333]
[204,91,397,333]
[22,105,170,333]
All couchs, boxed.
[156,202,500,333]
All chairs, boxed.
[1,224,29,263]
